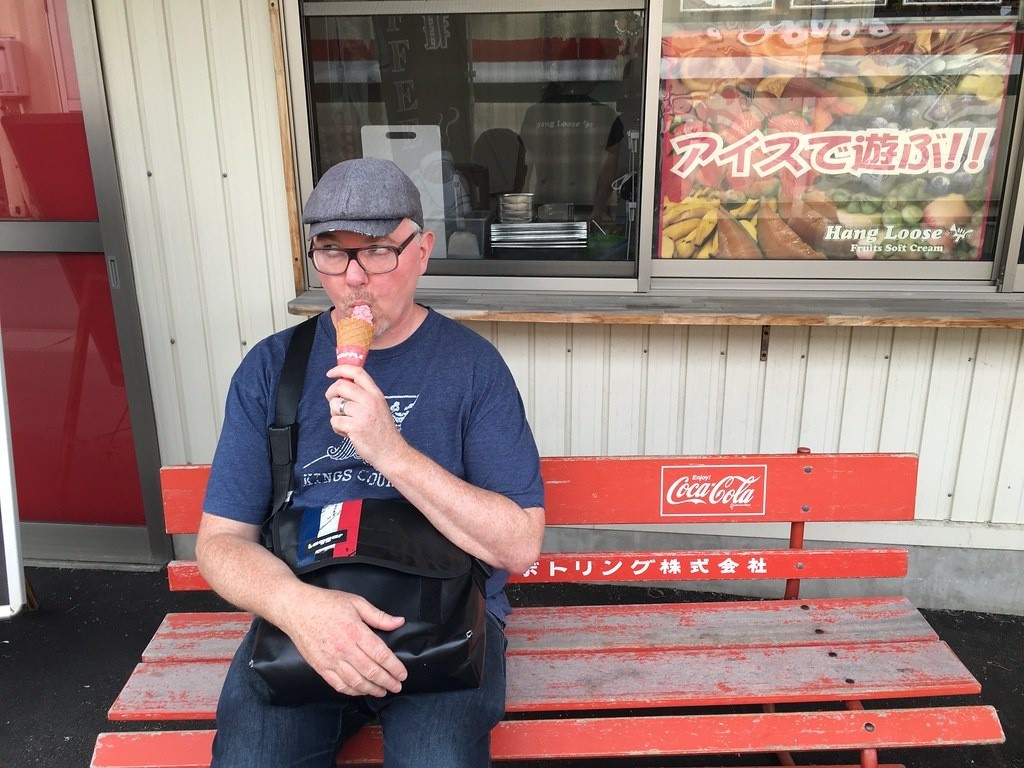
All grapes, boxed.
[812,101,1000,259]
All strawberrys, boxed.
[671,85,844,192]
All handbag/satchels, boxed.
[248,313,493,703]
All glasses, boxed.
[306,233,421,276]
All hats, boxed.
[301,157,424,242]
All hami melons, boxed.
[717,189,867,260]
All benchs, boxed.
[86,446,1004,767]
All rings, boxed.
[340,400,351,416]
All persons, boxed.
[195,157,545,768]
[458,128,585,262]
[583,57,644,235]
[521,35,624,231]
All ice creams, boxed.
[335,305,374,384]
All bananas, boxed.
[660,188,781,259]
